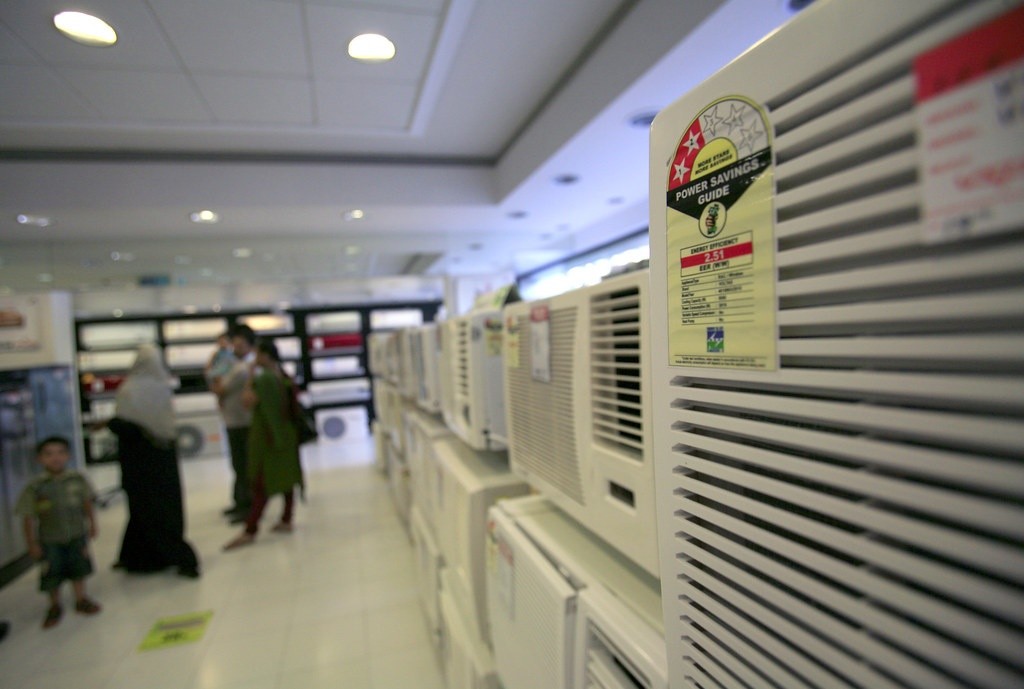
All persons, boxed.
[19,438,103,632]
[219,341,308,551]
[105,343,200,579]
[206,324,266,524]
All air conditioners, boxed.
[75,312,426,464]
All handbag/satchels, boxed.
[293,402,317,446]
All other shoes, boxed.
[179,567,199,577]
[44,605,62,627]
[224,508,246,523]
[0,622,9,640]
[77,600,99,613]
[225,532,254,550]
[272,520,293,533]
